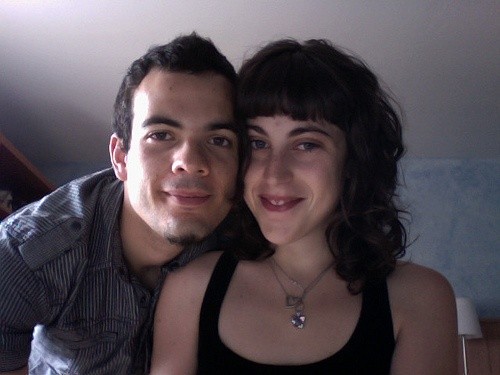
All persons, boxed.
[150,37,457,374]
[0,30,244,375]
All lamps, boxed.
[457,296,480,375]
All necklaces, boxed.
[266,254,336,331]
